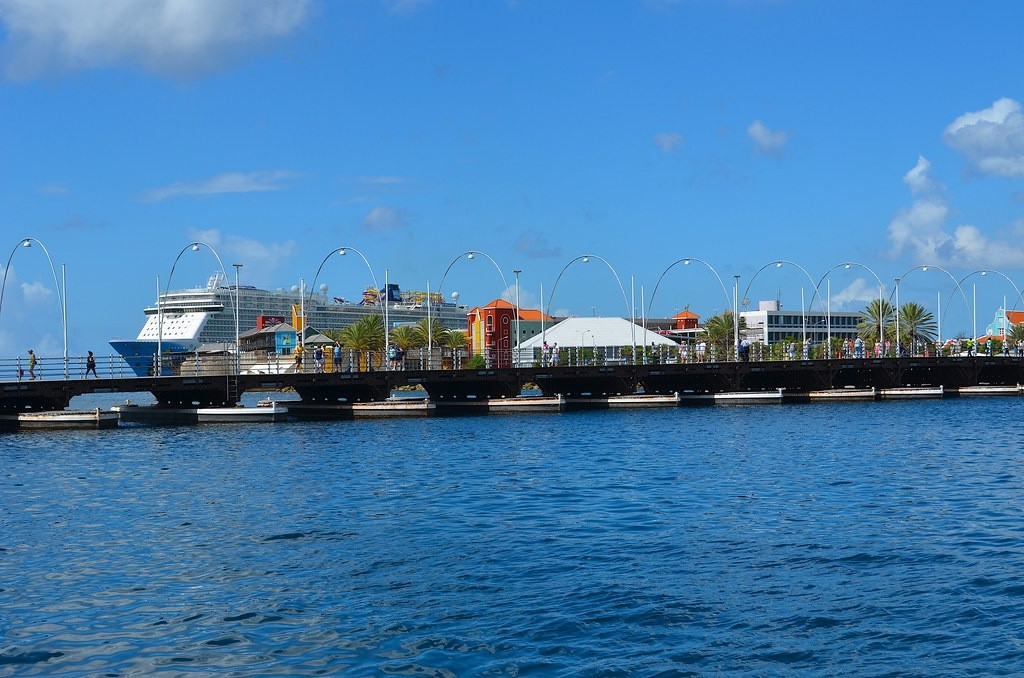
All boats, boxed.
[109,271,469,400]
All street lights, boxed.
[880,265,977,356]
[641,258,739,362]
[427,251,522,370]
[734,261,831,361]
[540,255,636,365]
[938,269,1024,357]
[1,237,70,380]
[156,242,243,377]
[801,262,900,358]
[301,247,391,371]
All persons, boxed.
[86,351,99,378]
[741,337,750,362]
[550,342,559,367]
[333,340,342,372]
[678,341,688,364]
[806,337,812,361]
[694,338,700,363]
[28,350,37,380]
[320,343,330,373]
[542,341,550,367]
[788,340,798,361]
[396,345,404,372]
[700,338,706,363]
[315,345,323,374]
[842,335,1024,359]
[389,345,396,369]
[738,337,744,362]
[293,341,310,374]
[652,342,658,364]
